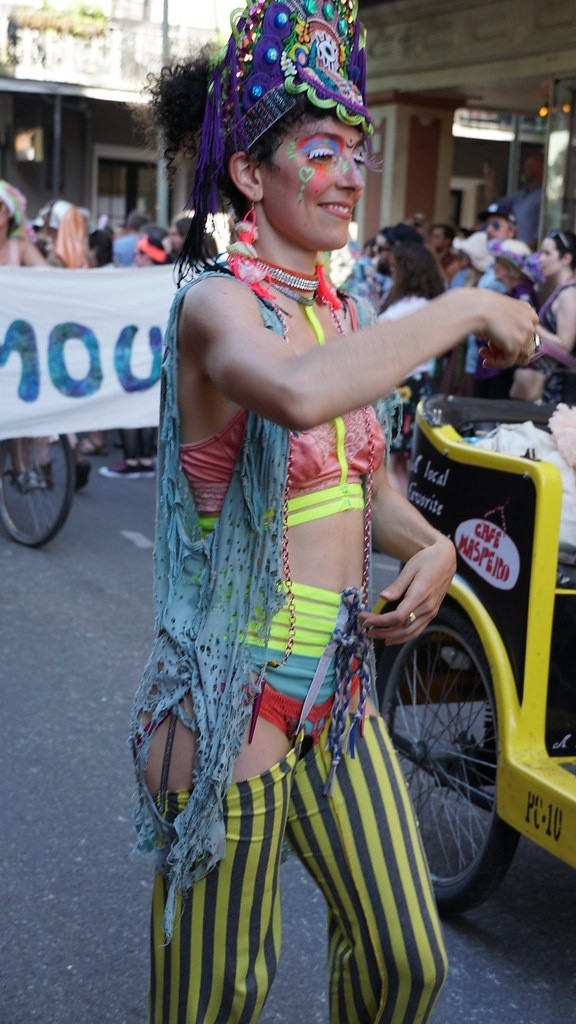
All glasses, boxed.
[373,241,390,252]
[480,221,510,230]
[548,229,569,248]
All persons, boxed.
[127,0,546,1024]
[1,176,576,494]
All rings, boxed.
[525,342,537,357]
[404,613,416,625]
[533,333,541,352]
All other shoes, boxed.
[98,462,141,479]
[35,464,54,489]
[140,464,155,477]
[73,461,91,492]
[75,443,107,456]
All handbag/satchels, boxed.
[486,421,576,546]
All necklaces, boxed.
[245,252,319,306]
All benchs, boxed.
[408,395,576,600]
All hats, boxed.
[176,218,192,237]
[120,213,149,231]
[33,200,74,229]
[452,231,497,272]
[0,179,27,226]
[486,237,546,284]
[477,200,517,224]
[387,224,424,245]
